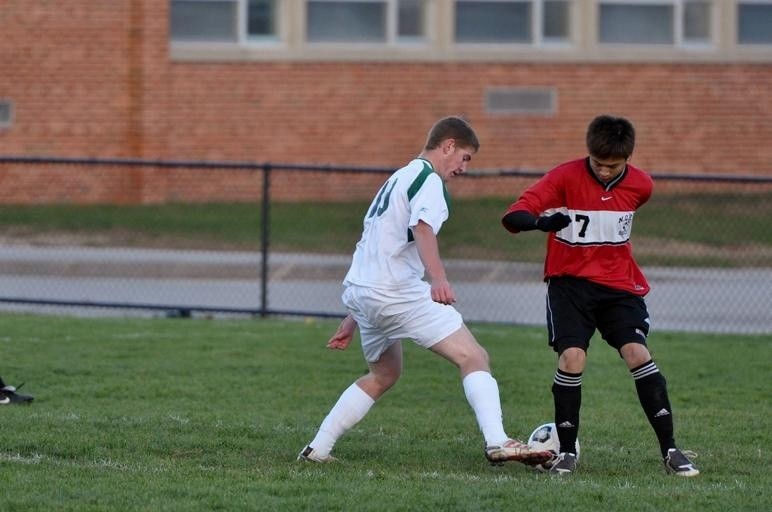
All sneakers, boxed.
[0,385,34,404]
[484,438,552,465]
[662,447,700,477]
[549,452,577,476]
[296,444,339,464]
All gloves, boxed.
[536,212,572,233]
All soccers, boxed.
[528,423,580,473]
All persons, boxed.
[1,376,34,407]
[296,115,553,468]
[500,115,703,478]
